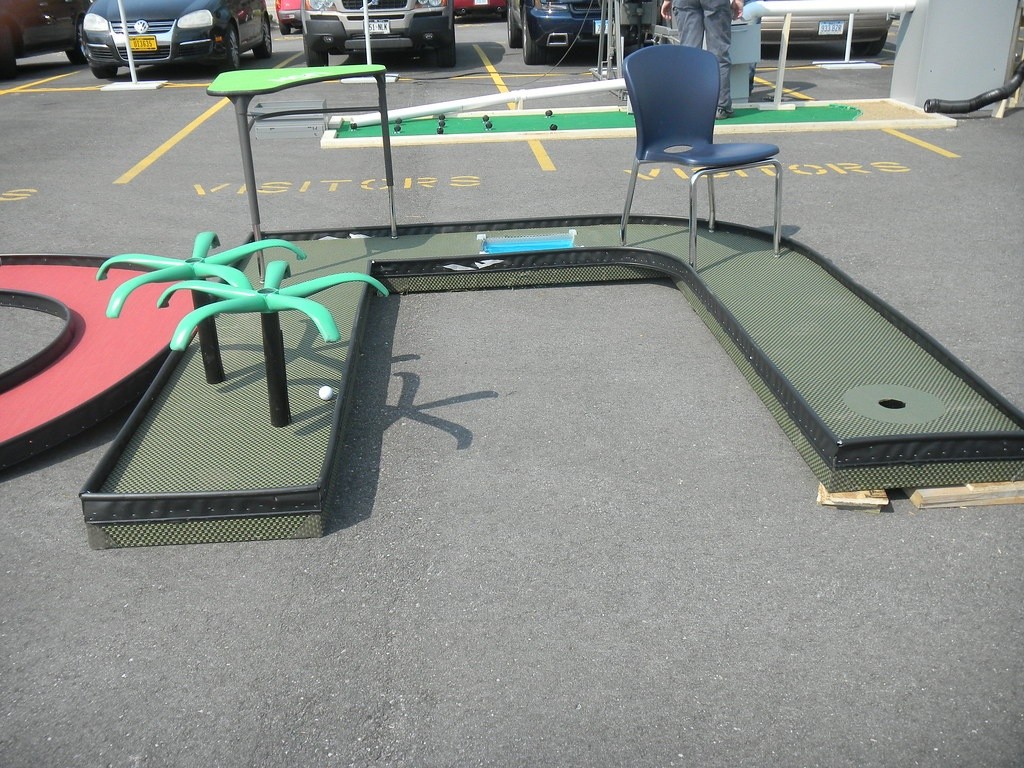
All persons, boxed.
[661,0,744,119]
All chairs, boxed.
[619,44,783,271]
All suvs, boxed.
[506,0,614,66]
[300,0,456,66]
[0,0,94,82]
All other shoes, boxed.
[715,106,734,119]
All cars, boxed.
[731,0,895,61]
[81,0,273,80]
[275,0,304,36]
[452,0,509,21]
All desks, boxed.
[205,64,399,284]
